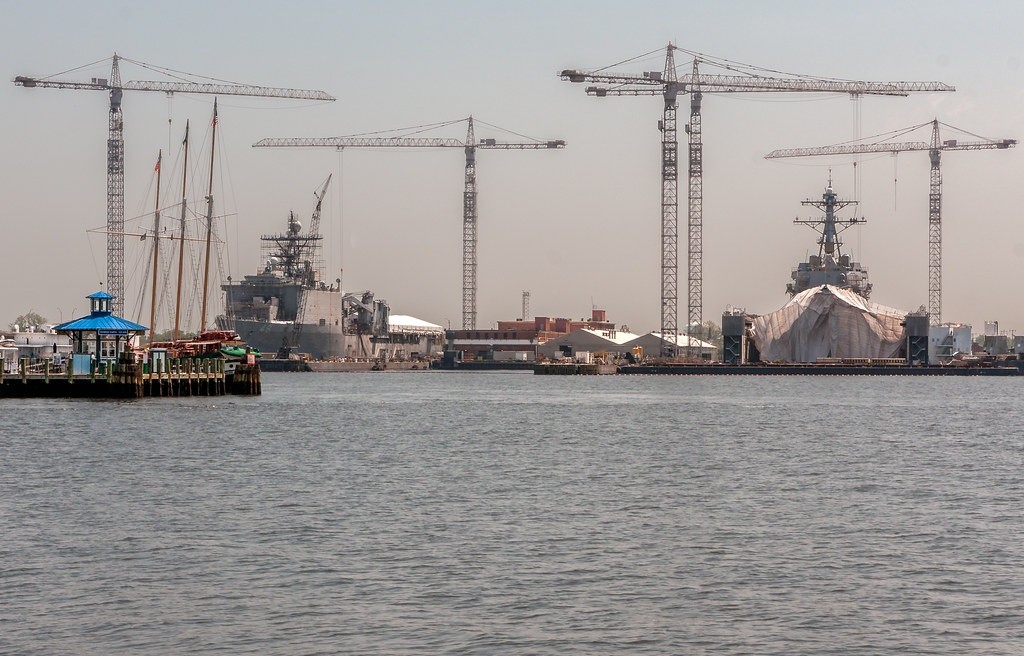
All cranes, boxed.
[247,105,568,343]
[13,53,337,338]
[556,37,1021,377]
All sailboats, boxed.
[124,95,259,379]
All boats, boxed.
[210,173,449,371]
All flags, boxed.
[154,151,161,172]
[211,102,218,128]
[182,120,188,144]
